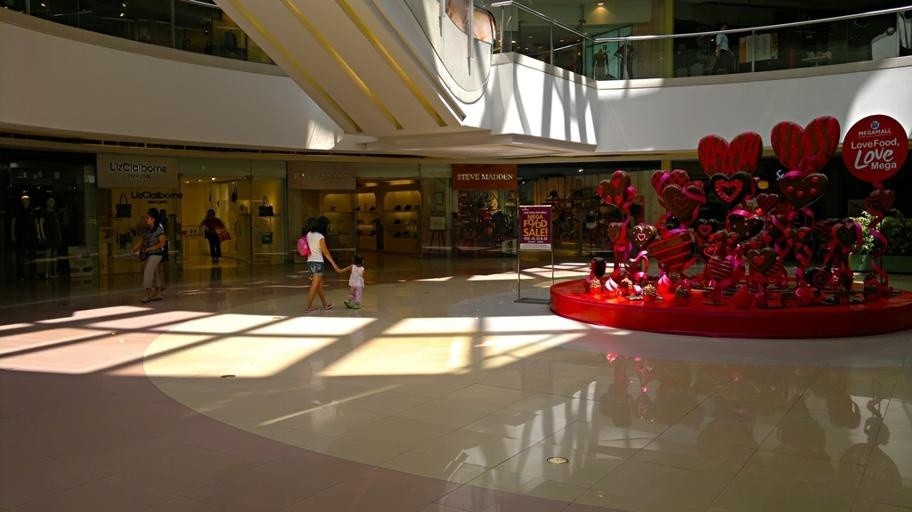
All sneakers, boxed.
[344,300,364,308]
[325,303,335,309]
[305,306,320,313]
[141,295,164,303]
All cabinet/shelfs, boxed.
[323,207,419,252]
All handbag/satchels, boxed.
[115,204,133,217]
[258,206,274,216]
[204,230,208,239]
[119,231,134,249]
[136,223,152,235]
[139,237,151,259]
[231,192,237,201]
[217,232,231,242]
[262,232,273,244]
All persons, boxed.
[149,208,170,292]
[591,48,609,80]
[614,44,635,79]
[201,209,224,263]
[304,215,340,312]
[11,194,42,280]
[340,254,366,309]
[711,22,735,74]
[131,211,163,303]
[39,197,59,279]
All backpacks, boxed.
[297,236,311,256]
